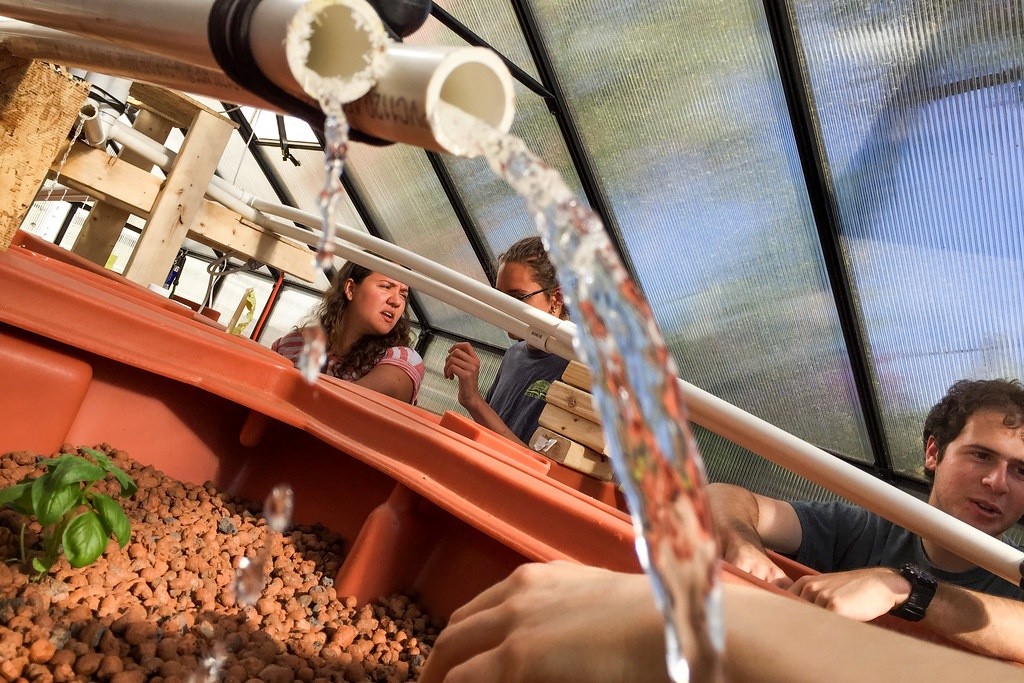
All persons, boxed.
[443,236,571,448]
[270,251,426,406]
[416,560,1023,683]
[706,378,1023,663]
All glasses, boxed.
[515,288,548,303]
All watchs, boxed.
[889,563,938,623]
